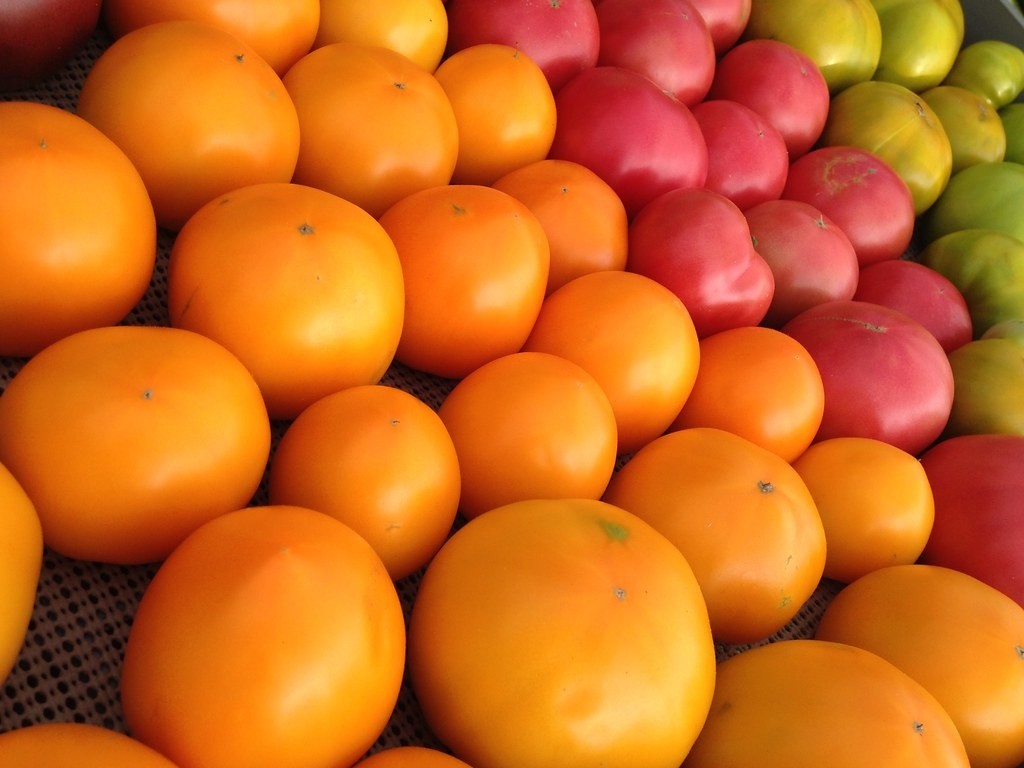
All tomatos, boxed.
[0,0,1024,766]
